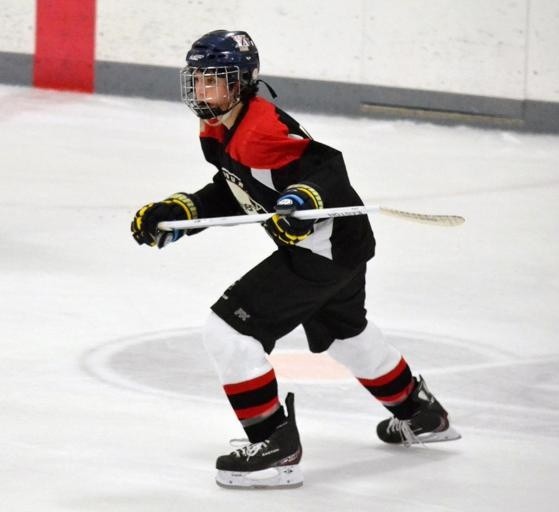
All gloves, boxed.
[261,184,323,248]
[130,190,200,251]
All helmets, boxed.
[177,28,262,120]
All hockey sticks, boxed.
[158,206,467,230]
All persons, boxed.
[130,31,449,473]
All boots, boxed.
[374,370,451,445]
[214,391,303,478]
